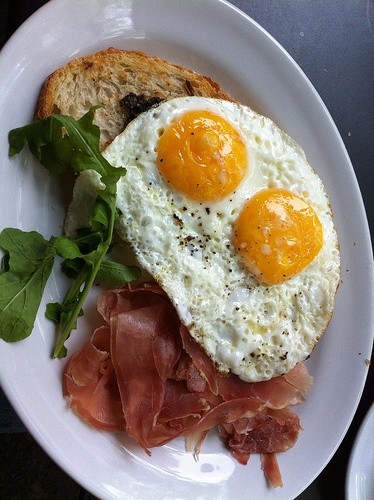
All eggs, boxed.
[95,96,340,383]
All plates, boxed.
[343,405,374,500]
[2,0,373,500]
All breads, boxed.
[35,49,236,152]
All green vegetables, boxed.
[0,103,140,360]
